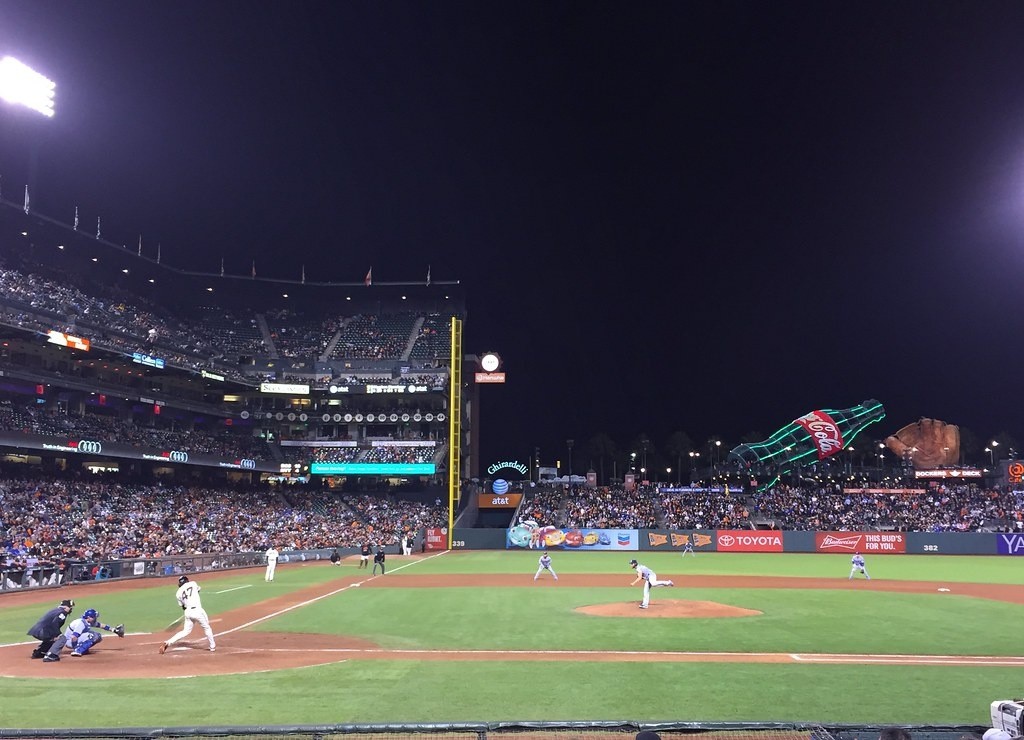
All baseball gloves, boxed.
[860,569,864,574]
[114,623,125,638]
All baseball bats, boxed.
[164,615,184,632]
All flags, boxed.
[156,243,161,264]
[426,266,431,287]
[22,186,30,215]
[252,262,256,277]
[72,209,79,231]
[365,269,372,287]
[220,258,225,275]
[97,218,101,239]
[138,235,141,254]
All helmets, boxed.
[178,575,189,586]
[83,609,99,620]
[58,599,75,608]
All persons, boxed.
[635,731,661,740]
[848,552,870,581]
[264,543,279,583]
[330,549,341,566]
[878,727,913,740]
[0,248,459,588]
[27,597,76,661]
[372,547,385,576]
[358,541,374,569]
[63,609,126,658]
[159,574,216,654]
[459,457,1024,532]
[533,551,558,582]
[628,560,674,609]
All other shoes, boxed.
[639,603,649,609]
[42,652,63,663]
[31,648,46,659]
[158,641,168,654]
[668,579,674,586]
[70,649,83,657]
[208,645,216,652]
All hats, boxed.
[543,551,548,555]
[855,551,860,556]
[379,547,384,550]
[629,559,637,564]
